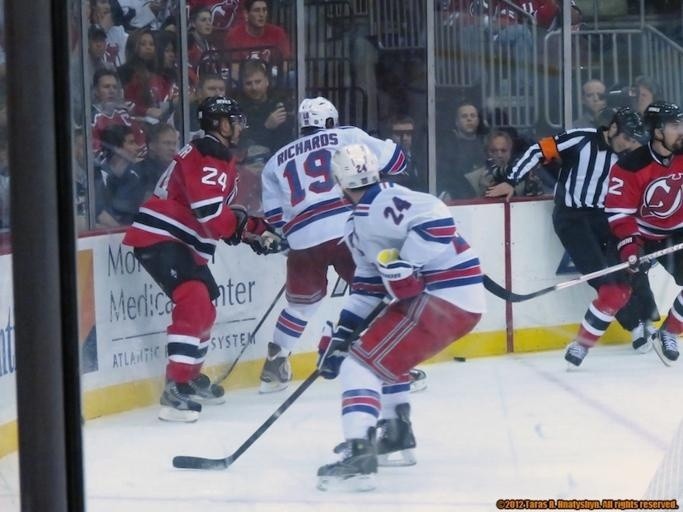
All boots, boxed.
[329,397,417,459]
[257,341,294,384]
[312,422,381,477]
[315,315,353,383]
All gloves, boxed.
[375,245,427,302]
[224,205,289,258]
[615,237,652,280]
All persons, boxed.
[316,144,485,477]
[71,0,663,230]
[485,106,661,349]
[121,94,275,412]
[563,101,683,366]
[260,96,427,384]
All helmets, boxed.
[326,141,382,192]
[295,95,338,131]
[196,94,242,123]
[611,107,648,140]
[642,101,681,125]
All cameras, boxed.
[486,158,510,185]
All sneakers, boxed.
[562,339,589,367]
[158,377,201,413]
[629,315,658,351]
[171,372,226,400]
[657,319,681,361]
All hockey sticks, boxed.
[482,241,683,303]
[173,294,392,470]
[213,280,285,386]
